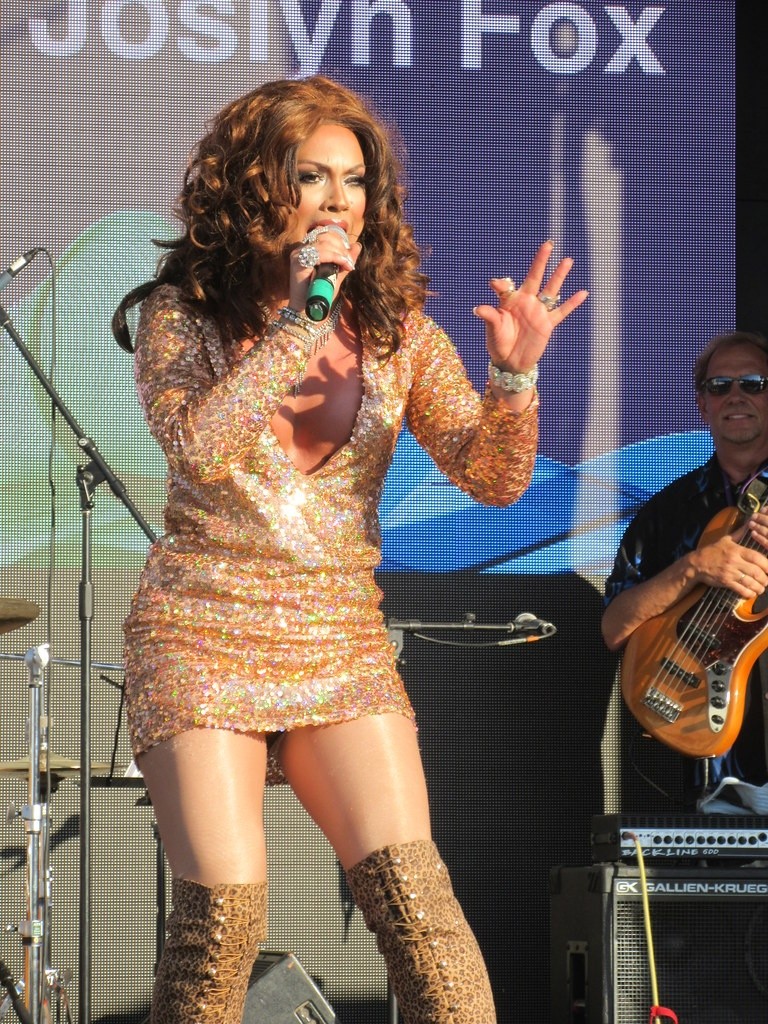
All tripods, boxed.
[0,644,73,1024]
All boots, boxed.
[150,879,268,1024]
[346,838,499,1024]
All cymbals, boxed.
[0,747,129,778]
[1,597,41,637]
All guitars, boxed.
[619,467,767,759]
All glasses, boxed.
[700,375,768,397]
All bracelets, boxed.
[486,360,539,393]
[273,305,316,344]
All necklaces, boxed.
[294,297,343,397]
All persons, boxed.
[122,78,591,1024]
[600,329,768,813]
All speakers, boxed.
[547,862,767,1024]
[239,950,343,1024]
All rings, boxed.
[496,277,514,298]
[302,225,327,243]
[297,246,320,267]
[539,291,557,308]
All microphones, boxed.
[514,613,556,638]
[0,247,38,291]
[306,224,349,323]
